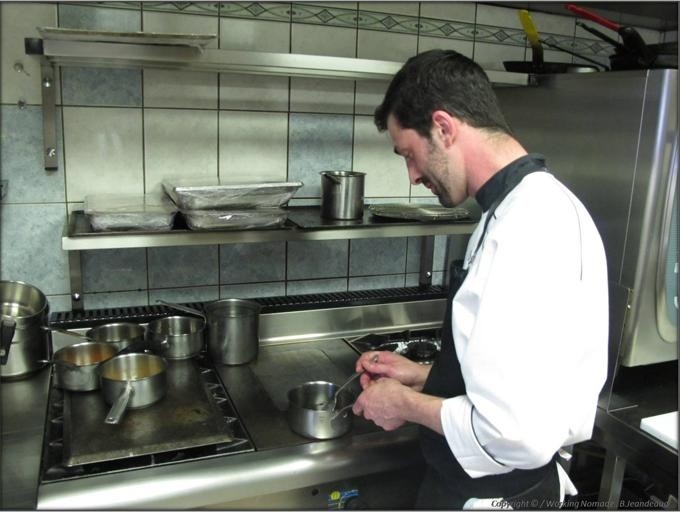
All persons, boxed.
[344,46,612,511]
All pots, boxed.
[502,1,678,71]
[287,381,358,442]
[0,278,260,428]
[320,167,364,221]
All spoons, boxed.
[320,355,380,416]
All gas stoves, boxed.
[349,329,450,362]
